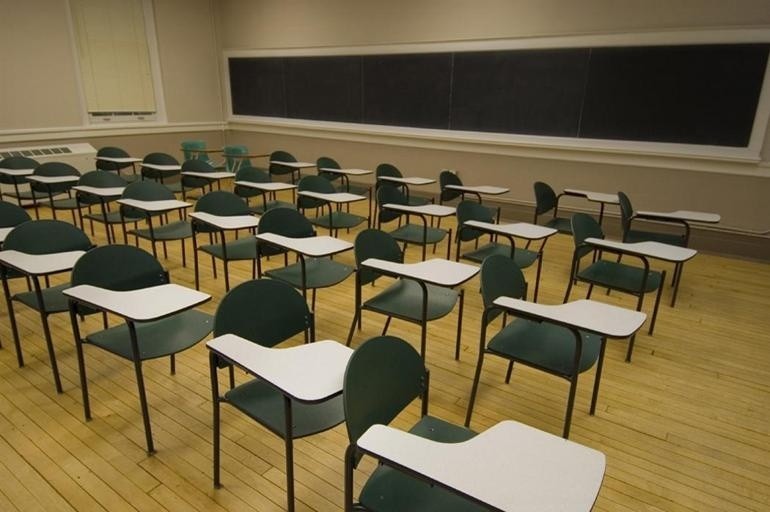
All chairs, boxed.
[268,151,316,203]
[0,219,110,395]
[439,169,511,203]
[185,190,288,292]
[455,200,557,303]
[137,152,183,223]
[376,163,438,225]
[376,185,457,262]
[179,139,226,191]
[615,189,722,286]
[179,159,236,221]
[115,180,201,267]
[466,254,648,439]
[73,170,131,244]
[95,146,142,212]
[346,228,479,363]
[1,156,40,205]
[297,175,368,260]
[342,334,605,512]
[563,212,697,363]
[0,200,50,291]
[233,166,299,240]
[317,155,374,234]
[205,277,357,512]
[255,206,362,342]
[62,243,236,455]
[221,144,272,172]
[26,161,95,235]
[523,178,620,285]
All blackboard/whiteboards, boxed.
[222,27,766,165]
